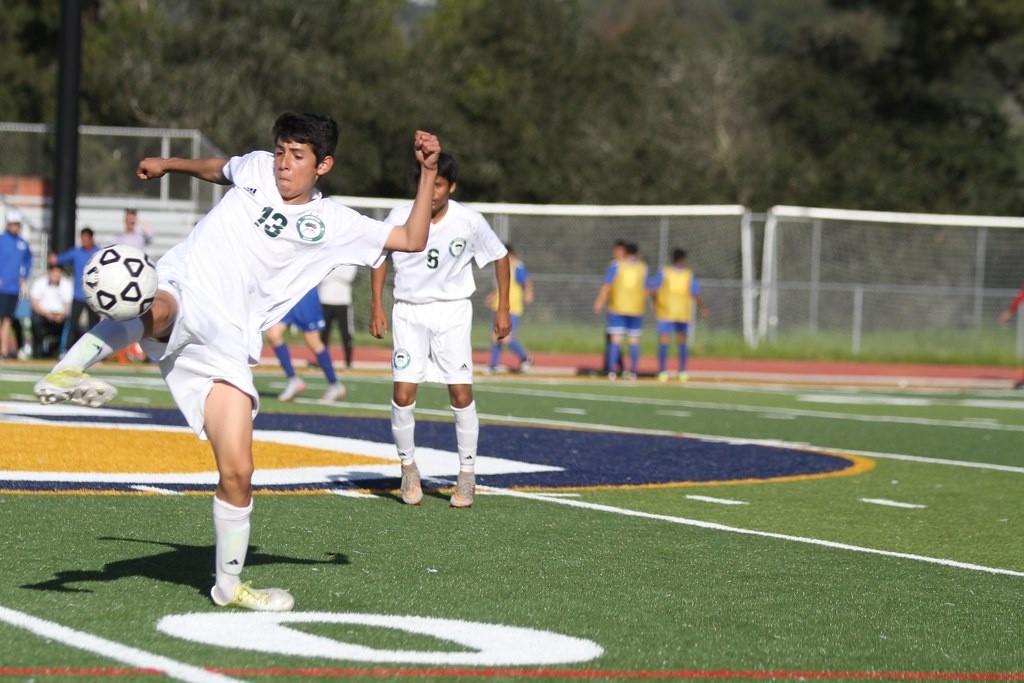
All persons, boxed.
[30,265,76,360]
[266,286,347,402]
[649,246,709,382]
[115,206,154,252]
[484,238,534,376]
[0,208,33,360]
[317,263,356,368]
[34,109,442,614]
[48,228,103,361]
[592,238,651,381]
[370,152,512,507]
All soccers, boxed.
[82,243,161,322]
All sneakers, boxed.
[211,580,295,612]
[449,472,475,508]
[317,381,347,404]
[33,368,119,408]
[400,463,424,505]
[278,375,307,403]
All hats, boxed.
[4,211,25,224]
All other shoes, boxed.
[482,367,498,376]
[596,370,615,378]
[676,371,689,383]
[655,372,668,384]
[626,372,638,379]
[516,356,535,372]
